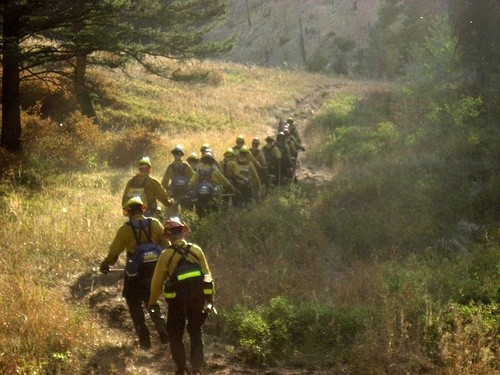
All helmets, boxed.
[201,144,213,158]
[251,138,260,144]
[276,117,293,136]
[223,148,234,157]
[239,146,249,154]
[236,135,245,143]
[125,196,143,207]
[161,216,188,239]
[137,156,151,166]
[186,152,200,161]
[265,135,274,140]
[171,145,184,157]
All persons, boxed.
[148,217,216,375]
[219,136,269,207]
[161,148,195,213]
[121,157,173,227]
[187,152,241,219]
[187,142,223,173]
[99,196,171,350]
[261,118,305,186]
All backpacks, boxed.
[126,178,148,210]
[169,243,204,308]
[127,217,164,288]
[194,166,216,204]
[169,163,188,197]
